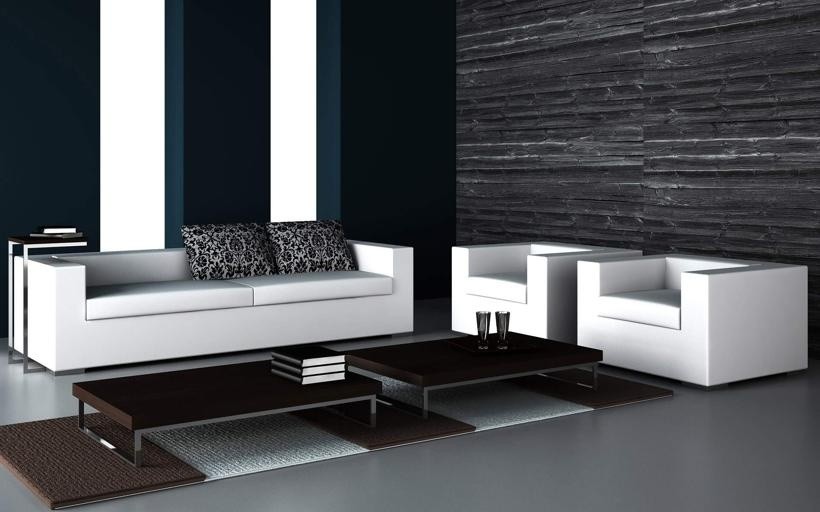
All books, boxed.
[270,368,347,385]
[29,232,83,239]
[271,346,347,367]
[271,358,348,378]
[40,226,77,234]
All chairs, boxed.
[573,251,809,391]
[449,242,643,349]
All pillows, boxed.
[268,220,358,274]
[174,223,276,280]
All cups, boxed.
[494,311,511,350]
[476,310,491,351]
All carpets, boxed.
[0,367,673,509]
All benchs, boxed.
[8,239,413,376]
